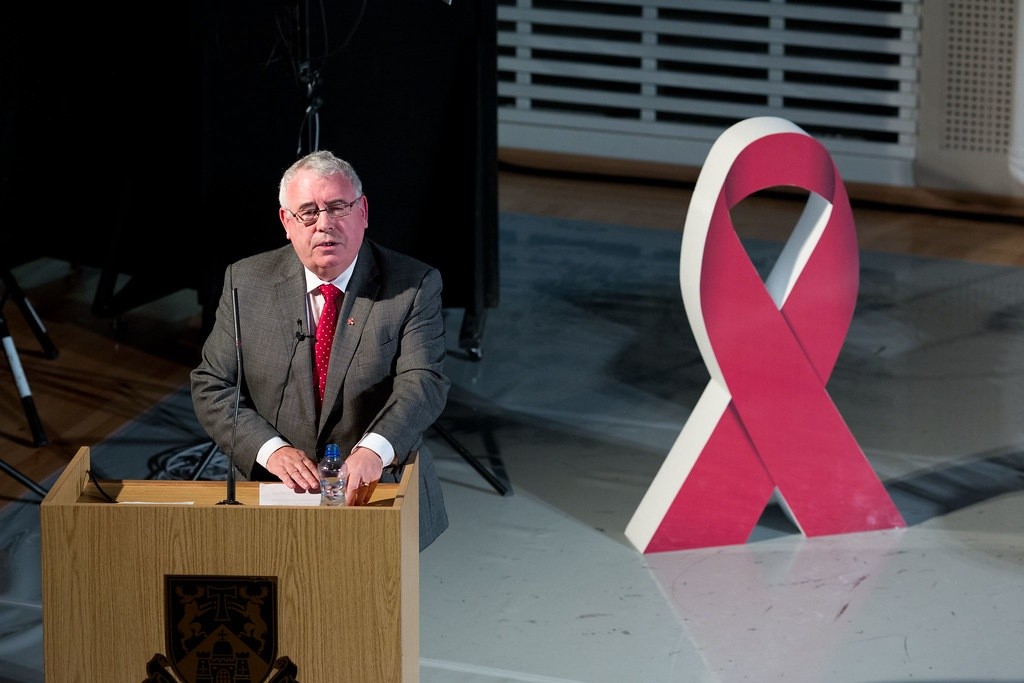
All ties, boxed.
[312,283,344,428]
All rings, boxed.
[361,481,371,487]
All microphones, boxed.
[215,287,242,507]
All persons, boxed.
[190,149,450,555]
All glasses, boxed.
[282,194,365,223]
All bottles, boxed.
[319,443,347,507]
